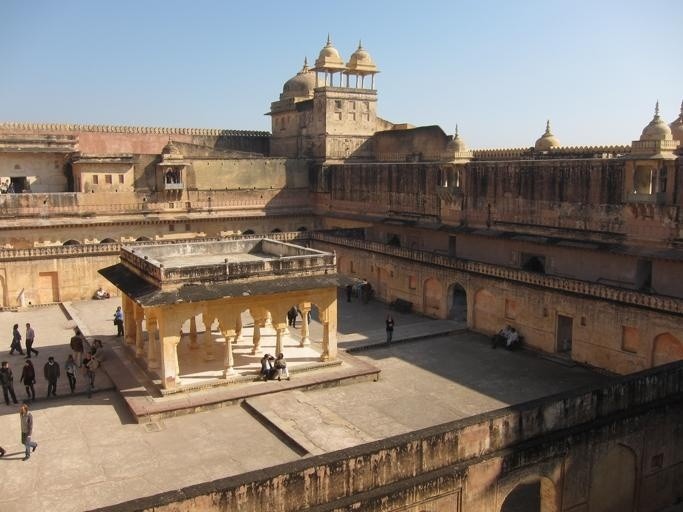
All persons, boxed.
[493,325,512,349]
[115,306,124,336]
[10,322,105,398]
[288,301,311,328]
[346,276,370,304]
[386,314,394,346]
[0,362,18,405]
[97,287,105,299]
[507,328,520,351]
[275,353,290,381]
[19,404,37,461]
[104,292,110,298]
[261,353,275,381]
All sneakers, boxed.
[264,377,290,381]
[33,444,37,451]
[0,450,5,456]
[23,456,29,461]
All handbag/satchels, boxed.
[277,365,286,368]
